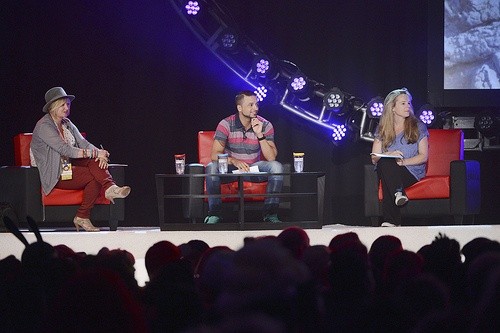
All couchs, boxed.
[364,128,482,226]
[182,131,292,225]
[0,132,129,231]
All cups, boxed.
[175,155,185,175]
[293,152,304,172]
[218,154,228,174]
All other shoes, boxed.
[263,215,281,223]
[381,222,396,227]
[394,192,408,206]
[204,216,219,224]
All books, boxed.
[370,153,403,159]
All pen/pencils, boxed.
[100,144,110,162]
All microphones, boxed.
[67,125,69,129]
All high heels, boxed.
[73,216,100,231]
[105,186,131,204]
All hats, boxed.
[43,86,76,113]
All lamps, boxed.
[366,96,386,123]
[322,86,354,118]
[252,54,281,84]
[182,0,208,20]
[473,109,500,145]
[287,71,316,103]
[416,103,438,127]
[220,32,246,58]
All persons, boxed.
[0,226,500,333]
[30,87,130,231]
[371,87,430,227]
[202,91,284,224]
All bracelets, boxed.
[257,134,266,141]
[84,147,98,159]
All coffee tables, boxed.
[154,171,328,231]
